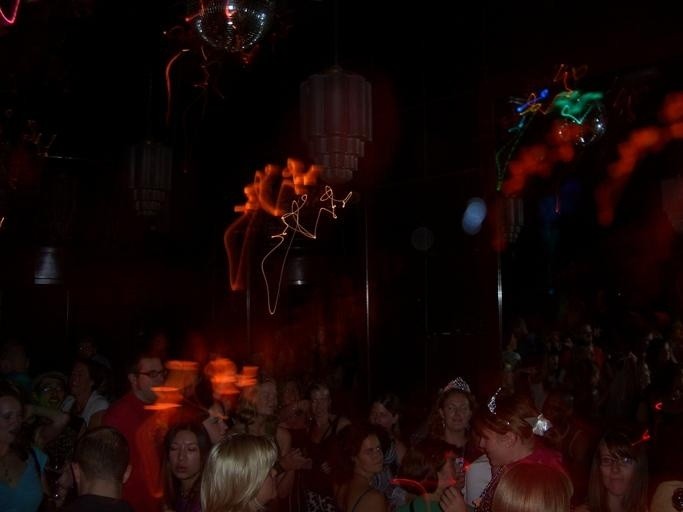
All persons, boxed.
[0,327,683,512]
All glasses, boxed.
[140,367,169,378]
